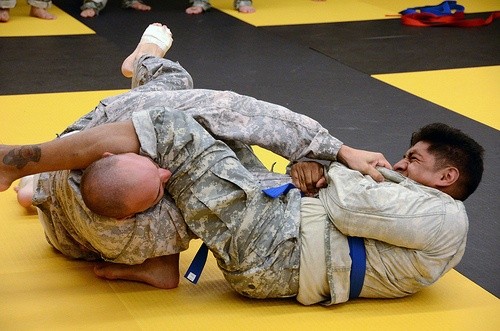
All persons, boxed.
[0,1,54,22]
[186,0,258,15]
[80,0,153,18]
[14,20,395,268]
[0,85,486,308]
